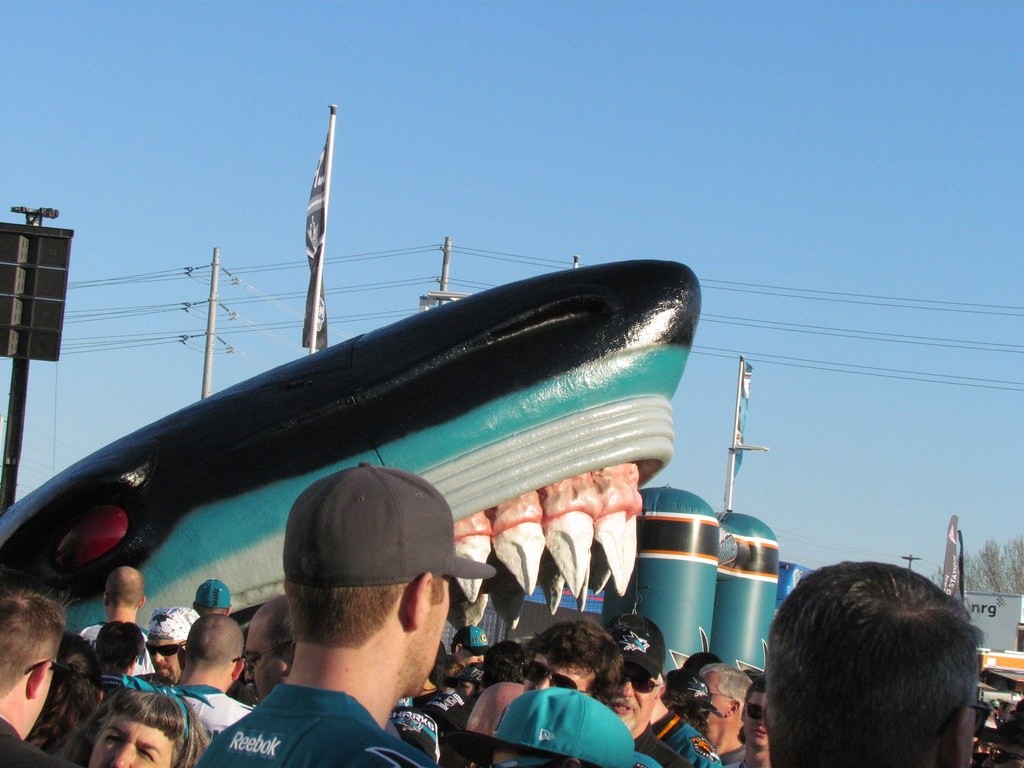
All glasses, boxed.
[973,742,1024,764]
[745,700,767,719]
[520,658,595,694]
[939,699,991,736]
[617,663,659,693]
[244,639,293,676]
[25,658,70,685]
[145,642,185,658]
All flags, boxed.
[302,130,329,351]
[942,516,968,605]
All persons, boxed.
[195,463,496,768]
[1,566,1024,768]
[602,613,690,768]
[764,561,979,768]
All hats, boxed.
[453,625,490,655]
[975,719,1024,747]
[602,614,665,681]
[990,699,1000,707]
[195,578,232,608]
[424,693,471,730]
[667,669,717,712]
[390,708,441,763]
[283,462,497,588]
[147,607,199,641]
[445,687,635,768]
[444,661,486,688]
